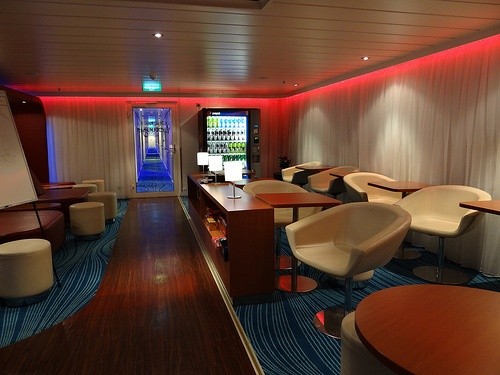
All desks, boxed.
[295,166,335,172]
[255,192,342,294]
[459,202,500,215]
[355,284,500,375]
[234,180,272,186]
[370,179,433,195]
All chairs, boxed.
[393,185,492,284]
[285,202,411,340]
[281,161,322,188]
[343,171,421,260]
[307,166,359,202]
[242,180,322,269]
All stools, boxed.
[73,178,105,194]
[88,192,117,224]
[0,238,53,308]
[68,202,105,240]
[340,312,392,375]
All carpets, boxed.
[230,228,500,375]
[0,199,129,348]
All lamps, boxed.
[224,161,243,199]
[208,156,222,184]
[196,152,209,176]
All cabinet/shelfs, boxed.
[187,173,276,299]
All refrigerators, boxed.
[198,107,261,179]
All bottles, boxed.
[207,128,245,140]
[221,155,247,170]
[206,116,245,128]
[207,141,246,153]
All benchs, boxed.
[0,182,88,254]
